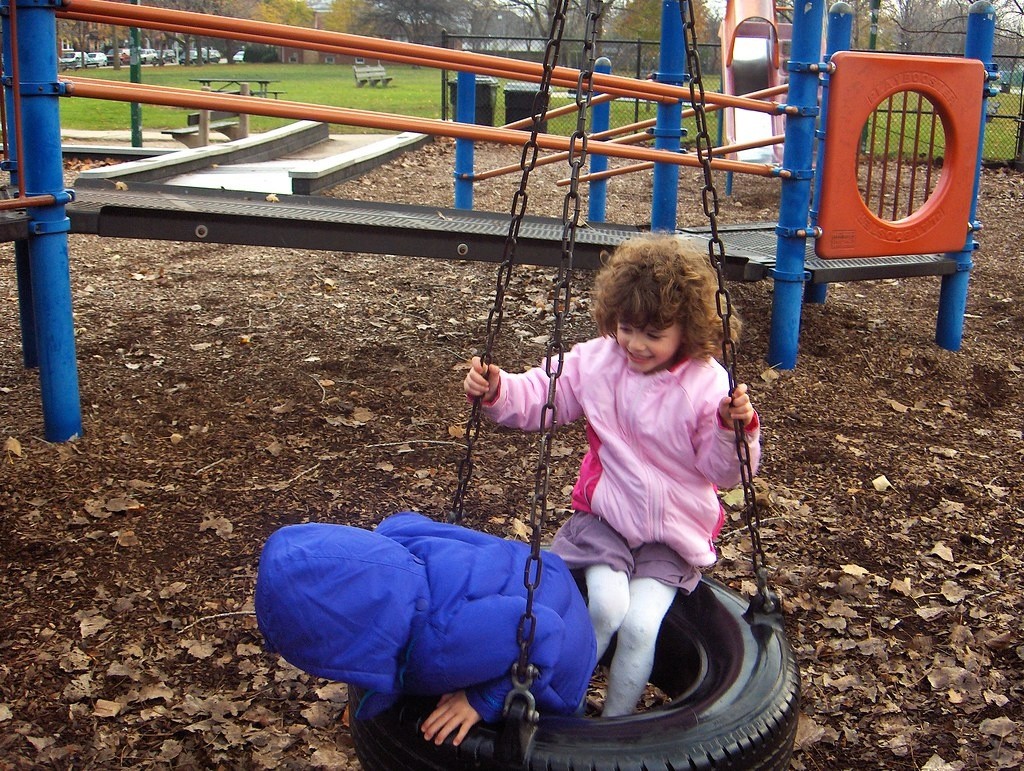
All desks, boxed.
[188,78,281,98]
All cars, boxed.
[59,44,245,69]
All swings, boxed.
[347,0,805,771]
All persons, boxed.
[465,228,762,716]
[255,512,597,748]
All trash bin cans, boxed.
[502,83,554,134]
[447,75,500,128]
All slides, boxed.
[718,36,784,166]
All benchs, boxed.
[85,62,99,69]
[202,89,287,100]
[352,63,393,87]
[60,62,79,71]
[151,58,164,66]
